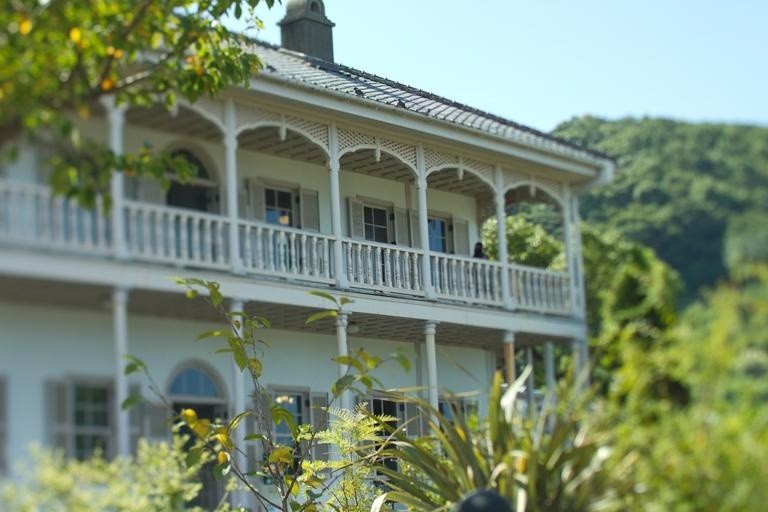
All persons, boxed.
[474,242,487,260]
